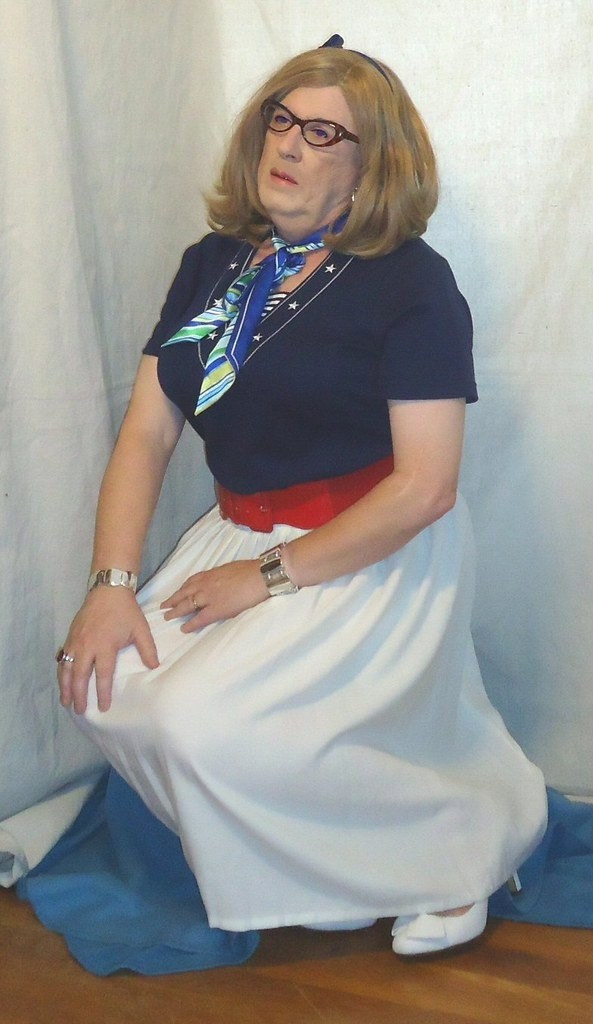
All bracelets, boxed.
[260,544,299,597]
[89,568,139,593]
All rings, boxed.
[55,648,74,664]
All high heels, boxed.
[391,869,521,955]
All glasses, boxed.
[259,98,360,147]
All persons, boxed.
[55,33,547,957]
